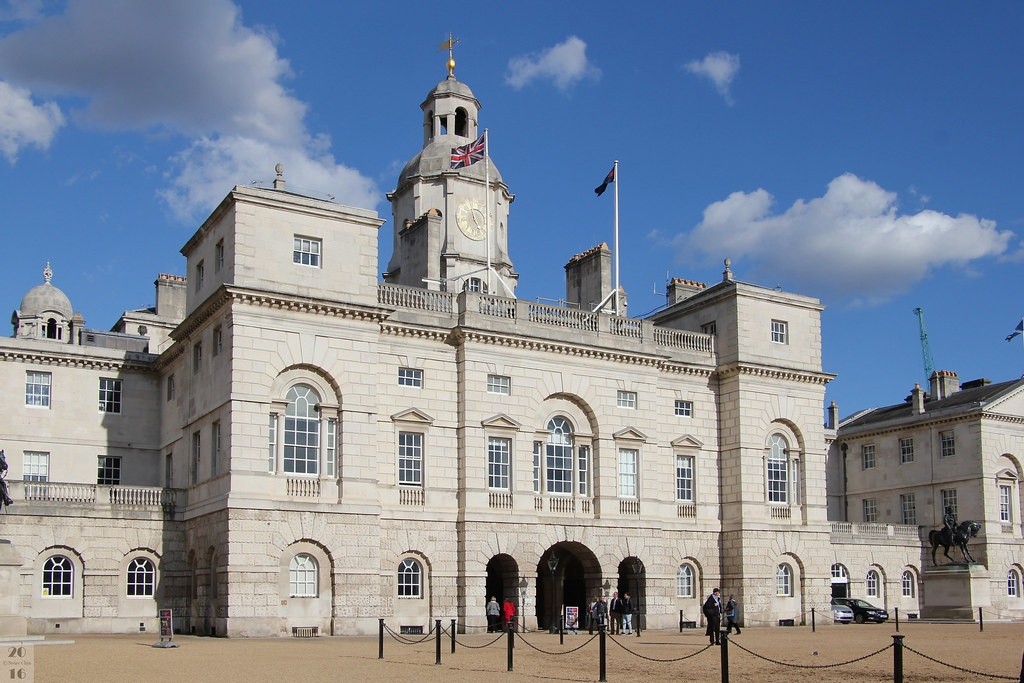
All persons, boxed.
[703,588,741,645]
[0,450,13,510]
[943,504,957,547]
[588,592,633,635]
[487,596,515,632]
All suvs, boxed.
[835,597,889,624]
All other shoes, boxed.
[716,643,721,645]
[734,632,741,635]
[628,633,632,634]
[621,632,625,634]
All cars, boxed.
[830,597,855,624]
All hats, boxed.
[729,593,735,600]
[713,588,720,592]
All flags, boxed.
[595,165,618,197]
[1005,320,1024,342]
[450,132,488,170]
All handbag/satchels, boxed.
[726,608,734,616]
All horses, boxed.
[928,519,982,567]
